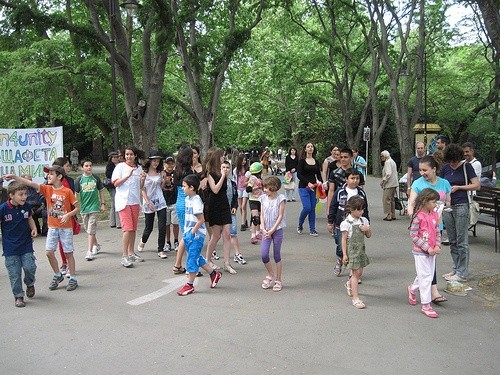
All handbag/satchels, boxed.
[73,216,81,235]
[394,191,403,210]
[470,201,479,225]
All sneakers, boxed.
[297,224,303,234]
[177,283,194,296]
[66,267,71,277]
[60,265,69,275]
[421,306,438,317]
[92,244,101,254]
[210,271,222,288]
[164,244,170,252]
[174,242,178,251]
[240,220,249,231]
[67,279,78,291]
[26,286,35,297]
[15,297,25,307]
[212,251,219,260]
[309,230,318,236]
[408,285,417,305]
[234,254,246,264]
[49,273,64,290]
[128,253,144,262]
[121,257,133,266]
[85,252,96,259]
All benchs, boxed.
[399,182,500,252]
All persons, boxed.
[481,170,497,187]
[437,142,481,282]
[201,146,262,274]
[70,145,79,171]
[177,174,222,296]
[74,158,106,261]
[442,143,482,245]
[296,142,323,237]
[380,150,399,221]
[327,168,370,284]
[1,164,81,292]
[322,145,366,215]
[407,187,441,317]
[399,136,451,216]
[257,177,286,292]
[43,157,80,278]
[163,143,209,278]
[408,155,452,303]
[0,180,38,307]
[339,195,372,309]
[138,151,167,259]
[105,146,146,267]
[286,147,297,202]
[249,145,284,176]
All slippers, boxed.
[432,295,448,304]
[383,217,396,221]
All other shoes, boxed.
[445,281,471,296]
[286,197,290,202]
[256,235,262,240]
[444,275,467,281]
[250,238,258,243]
[292,196,296,201]
[442,271,454,277]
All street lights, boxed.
[362,125,370,175]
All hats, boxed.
[146,151,164,159]
[249,162,263,174]
[166,157,174,162]
[43,165,65,178]
[108,152,120,157]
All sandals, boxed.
[158,253,167,258]
[262,276,273,288]
[334,258,343,276]
[209,261,220,270]
[138,238,146,252]
[352,300,367,308]
[344,281,352,296]
[224,265,238,274]
[172,265,186,274]
[186,269,203,277]
[272,280,282,291]
